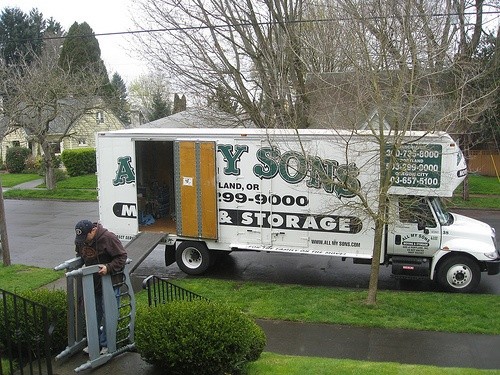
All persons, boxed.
[74,220,128,356]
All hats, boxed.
[74,219,94,242]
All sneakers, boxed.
[83,343,88,354]
[100,346,109,356]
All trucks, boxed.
[92,126,499,293]
